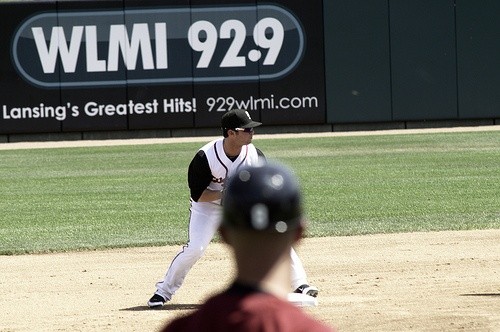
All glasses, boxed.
[234,127,254,133]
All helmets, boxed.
[222,165,302,234]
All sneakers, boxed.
[295,285,319,297]
[147,295,165,308]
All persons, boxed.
[147,108,319,308]
[159,160,333,332]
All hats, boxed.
[221,109,262,133]
